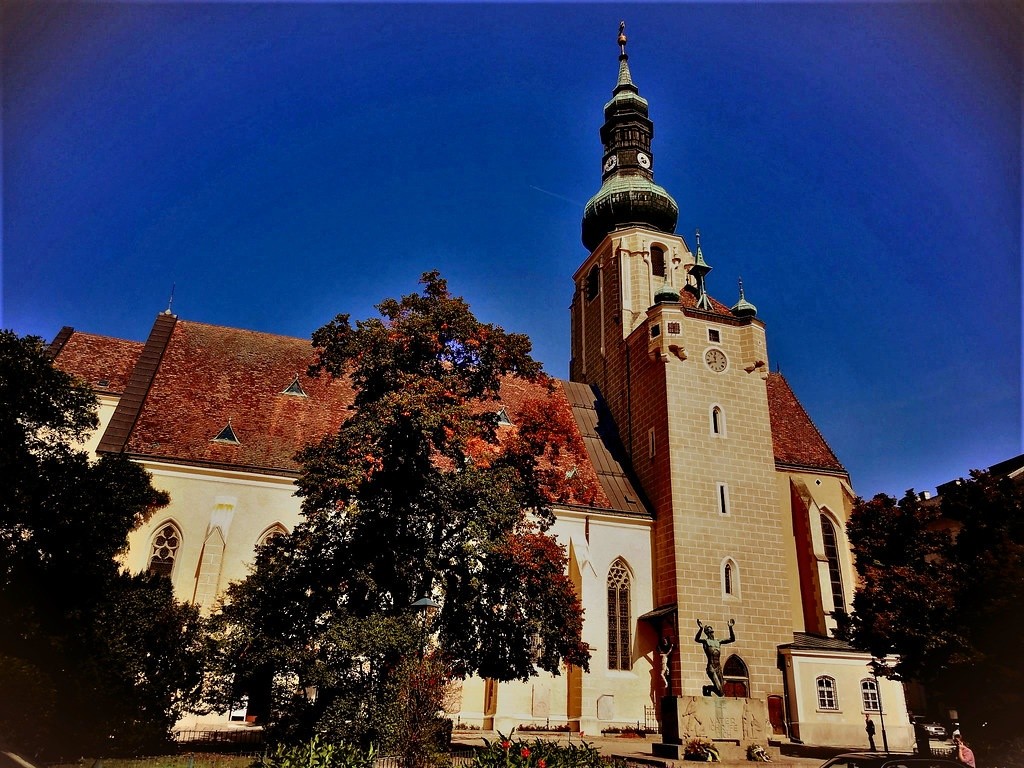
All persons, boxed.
[695,618,736,697]
[658,644,674,689]
[865,713,877,752]
[951,734,975,768]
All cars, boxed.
[820,752,974,768]
[952,723,960,741]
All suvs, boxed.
[910,715,948,741]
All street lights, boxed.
[866,658,888,752]
[409,592,440,664]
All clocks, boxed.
[605,155,616,170]
[637,153,650,168]
[705,349,728,369]
